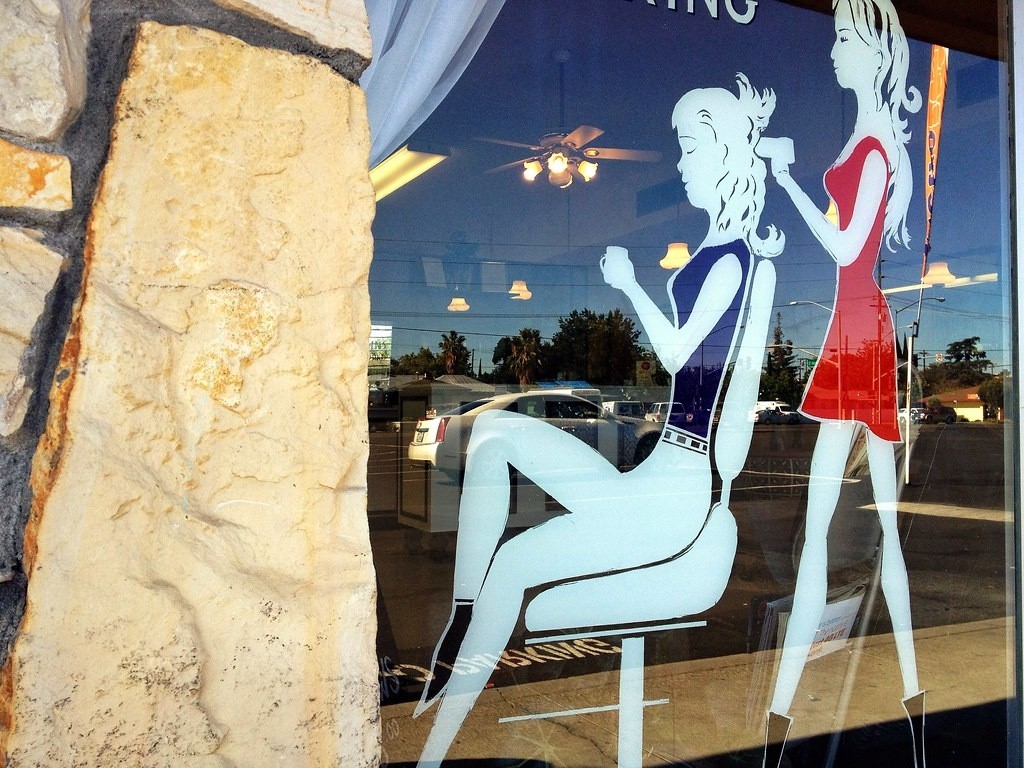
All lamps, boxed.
[659,146,691,269]
[447,297,470,311]
[919,262,957,285]
[507,280,532,300]
[523,142,599,189]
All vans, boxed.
[898,404,958,425]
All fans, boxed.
[472,48,663,174]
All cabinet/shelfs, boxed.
[746,359,924,768]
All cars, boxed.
[687,408,710,423]
[755,408,797,426]
[712,403,722,427]
[403,391,663,486]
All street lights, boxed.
[697,322,743,393]
[892,296,946,335]
[789,299,844,424]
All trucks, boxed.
[545,389,599,406]
[602,400,642,416]
[757,401,799,425]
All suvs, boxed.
[644,401,686,425]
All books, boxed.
[746,420,922,735]
